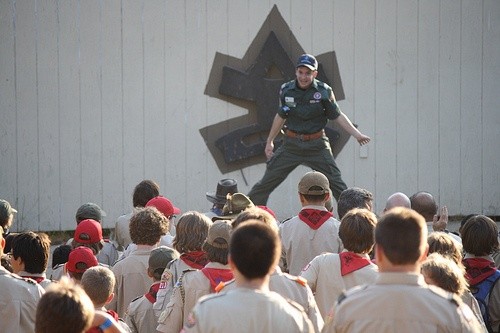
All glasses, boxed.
[7,252,14,260]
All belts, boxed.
[286,129,326,142]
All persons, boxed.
[121,197,180,257]
[321,207,484,333]
[81,266,132,333]
[183,219,315,333]
[248,54,370,205]
[0,199,21,273]
[421,230,488,333]
[156,221,231,333]
[7,232,51,290]
[459,214,500,271]
[103,206,169,319]
[50,219,110,280]
[52,203,118,269]
[112,180,159,251]
[297,207,378,324]
[153,210,213,333]
[127,246,181,333]
[35,281,128,333]
[209,170,448,277]
[0,226,46,333]
[461,215,500,333]
[67,246,98,280]
[231,208,324,333]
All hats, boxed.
[73,219,104,244]
[0,200,17,227]
[298,169,330,196]
[75,203,106,222]
[66,247,98,274]
[206,219,233,249]
[148,246,181,278]
[296,54,319,72]
[206,179,239,204]
[145,197,180,218]
[212,193,256,223]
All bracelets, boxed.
[100,317,115,329]
